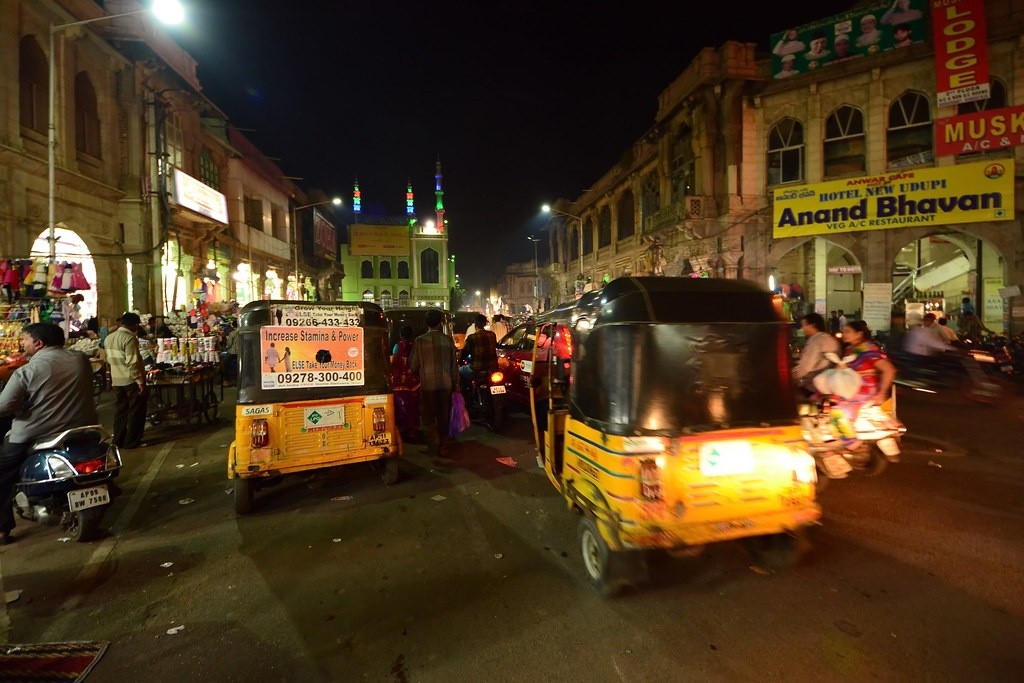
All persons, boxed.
[790,297,998,381]
[809,320,896,448]
[0,322,97,544]
[409,309,459,453]
[773,54,800,80]
[104,313,149,449]
[772,28,807,57]
[854,14,882,48]
[803,31,830,60]
[880,0,922,26]
[789,312,840,403]
[456,313,500,405]
[826,33,864,68]
[889,23,913,50]
[68,315,240,391]
[464,316,533,346]
[279,347,291,372]
[265,343,279,372]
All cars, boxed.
[496,321,537,406]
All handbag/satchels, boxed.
[448,389,471,437]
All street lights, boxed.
[540,204,585,275]
[291,197,341,296]
[47,0,187,268]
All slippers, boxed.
[0,536,14,544]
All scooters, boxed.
[13,424,124,542]
[464,368,512,434]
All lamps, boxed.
[200,277,219,285]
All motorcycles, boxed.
[788,324,1024,476]
[224,299,403,516]
[529,275,821,600]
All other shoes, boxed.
[438,447,449,458]
